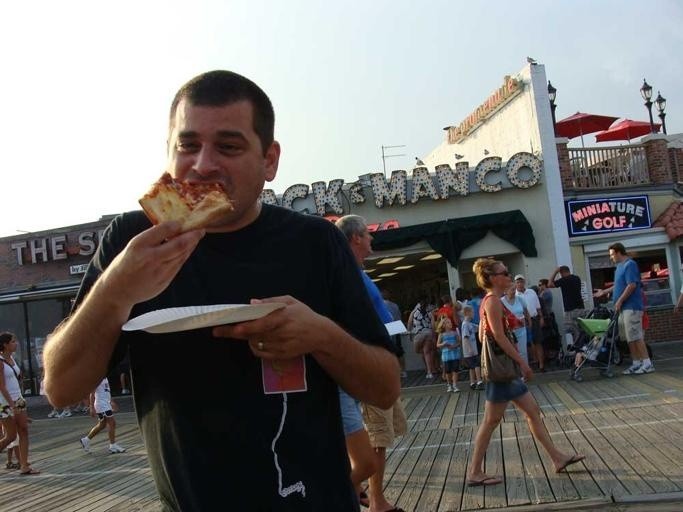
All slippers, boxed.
[556,453,585,472]
[468,473,500,486]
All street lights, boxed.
[639,77,655,134]
[546,79,557,135]
[653,90,667,134]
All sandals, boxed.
[6,461,39,475]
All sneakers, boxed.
[48,410,72,418]
[79,437,91,453]
[621,363,656,374]
[426,373,484,393]
[108,447,125,453]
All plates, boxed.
[119,304,283,332]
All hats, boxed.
[515,275,525,280]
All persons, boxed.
[335,214,409,512]
[468,258,585,487]
[0,331,130,475]
[593,242,656,374]
[41,70,402,512]
[675,287,683,313]
[503,266,585,378]
[407,288,485,393]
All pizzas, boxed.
[138,171,235,242]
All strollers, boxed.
[608,312,653,365]
[563,307,618,381]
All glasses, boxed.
[487,270,508,276]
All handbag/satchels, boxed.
[480,334,522,383]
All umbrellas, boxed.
[555,110,621,174]
[595,117,662,145]
